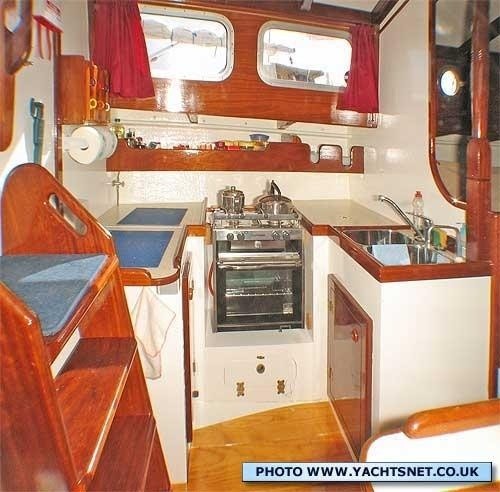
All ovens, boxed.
[214,230,306,331]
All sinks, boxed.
[342,227,415,246]
[366,245,457,268]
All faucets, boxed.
[427,225,461,257]
[378,195,425,241]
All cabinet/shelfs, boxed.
[0,161,172,492]
[326,272,368,462]
[54,55,110,125]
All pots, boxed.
[259,179,294,226]
[222,186,244,210]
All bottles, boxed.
[412,191,424,230]
[109,119,125,139]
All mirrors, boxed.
[429,0,500,213]
